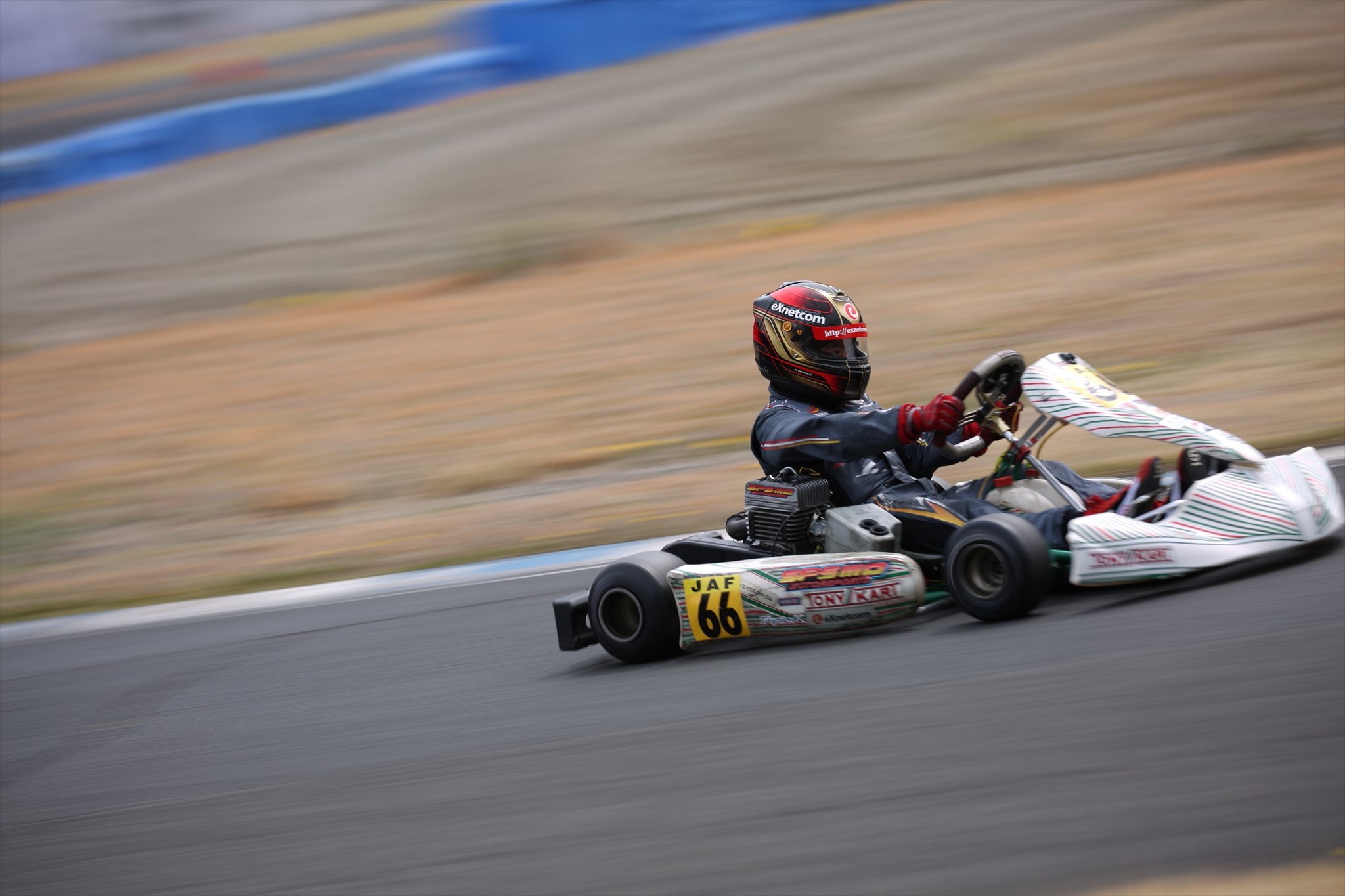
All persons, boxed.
[751,281,1211,549]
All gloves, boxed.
[962,401,1023,457]
[898,394,965,446]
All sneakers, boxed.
[1169,448,1210,504]
[1085,458,1162,518]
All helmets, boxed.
[752,280,871,401]
